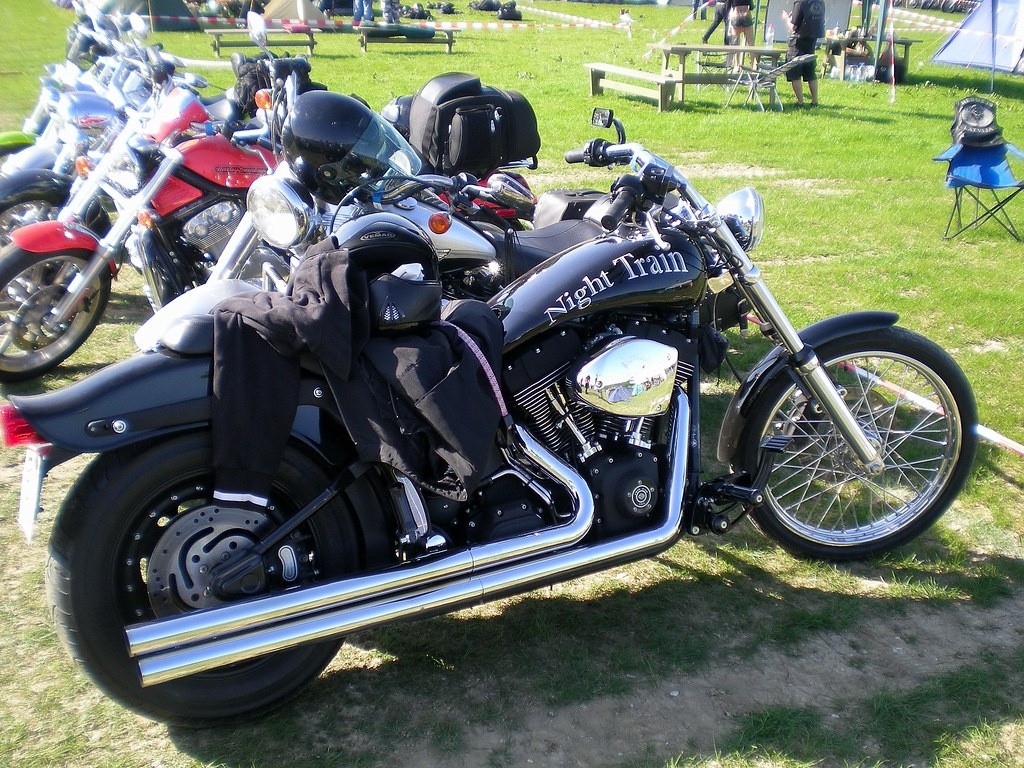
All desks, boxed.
[646,42,788,105]
[821,36,923,83]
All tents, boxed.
[929,0,1024,73]
[260,0,325,20]
[91,0,200,31]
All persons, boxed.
[353,0,372,21]
[692,0,707,20]
[786,0,826,107]
[378,0,401,24]
[238,0,264,28]
[702,0,755,68]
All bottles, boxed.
[766,24,775,49]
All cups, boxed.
[845,30,851,38]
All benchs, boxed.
[353,26,461,54]
[204,28,322,56]
[583,62,683,112]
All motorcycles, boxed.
[2,109,974,732]
[1,0,612,428]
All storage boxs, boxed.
[829,54,878,82]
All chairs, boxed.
[697,45,739,93]
[933,95,1024,242]
[725,54,819,113]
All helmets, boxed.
[332,210,442,335]
[281,89,379,206]
